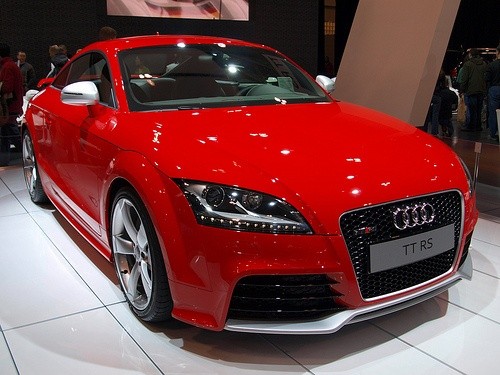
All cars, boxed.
[19,34,481,337]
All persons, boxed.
[0,41,70,167]
[98,26,118,41]
[427,45,500,139]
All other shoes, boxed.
[488,135,496,140]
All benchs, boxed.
[95,75,226,104]
